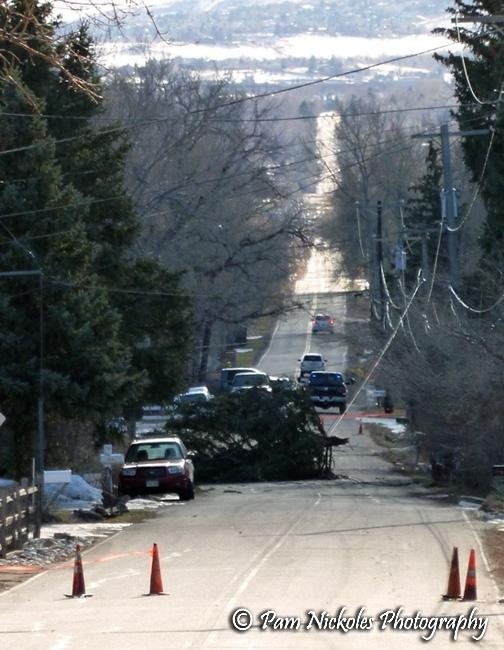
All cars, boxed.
[118,437,196,501]
[310,312,335,336]
[172,353,348,415]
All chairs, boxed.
[136,448,176,458]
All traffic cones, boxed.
[440,547,478,602]
[141,542,169,596]
[64,544,93,598]
[357,418,364,435]
